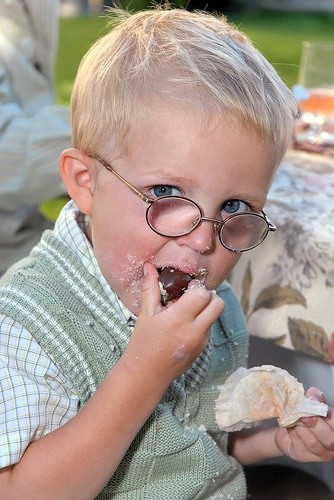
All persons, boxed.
[0,0,334,500]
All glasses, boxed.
[85,151,277,254]
